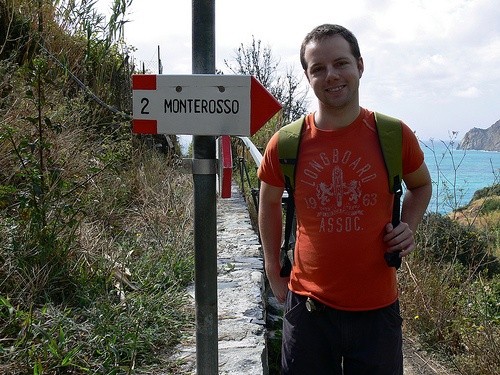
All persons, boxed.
[257,23,432,375]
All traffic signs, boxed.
[131,73,285,137]
